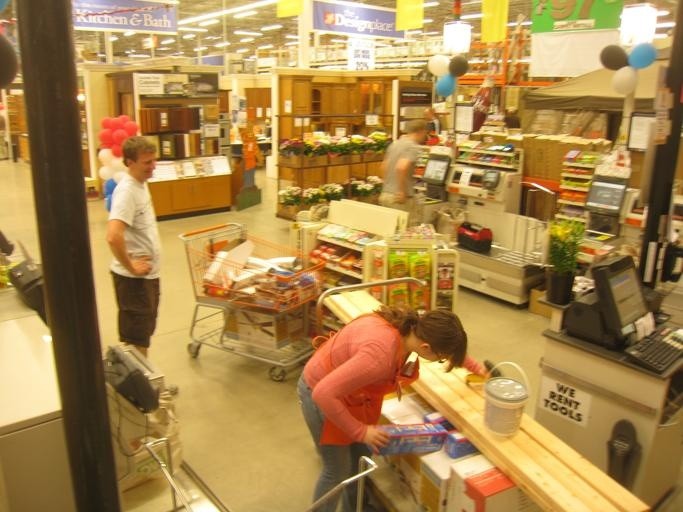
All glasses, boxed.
[433,350,446,363]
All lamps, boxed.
[443,0,471,54]
[620,0,657,45]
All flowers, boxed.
[278,174,384,206]
[546,220,586,271]
[278,131,393,159]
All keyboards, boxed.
[625,327,683,374]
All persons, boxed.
[375,119,429,227]
[105,136,163,358]
[423,108,441,135]
[296,302,490,511]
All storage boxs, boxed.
[224,309,305,343]
[528,282,552,319]
[378,389,541,512]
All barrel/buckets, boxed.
[483,361,530,438]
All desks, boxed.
[536,281,682,511]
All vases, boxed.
[546,269,575,305]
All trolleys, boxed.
[177,223,326,382]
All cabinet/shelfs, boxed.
[315,278,650,511]
[275,74,399,221]
[0,284,183,512]
[302,221,386,342]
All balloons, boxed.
[626,42,657,70]
[448,54,468,77]
[433,73,456,96]
[426,54,451,77]
[599,43,629,70]
[612,65,637,94]
[97,113,138,212]
